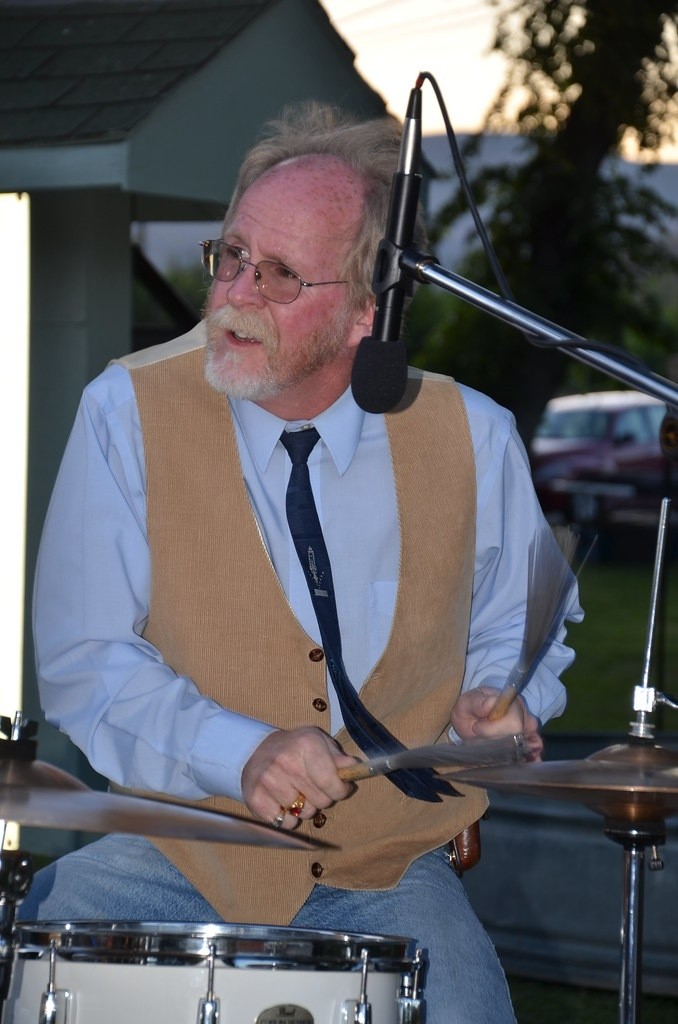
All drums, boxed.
[0,919,432,1022]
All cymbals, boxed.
[0,756,322,850]
[432,742,678,846]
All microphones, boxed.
[349,89,424,414]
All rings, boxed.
[286,793,305,817]
[271,809,287,830]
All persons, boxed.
[0,96,589,1024]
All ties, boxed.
[279,427,465,803]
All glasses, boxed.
[199,238,348,304]
